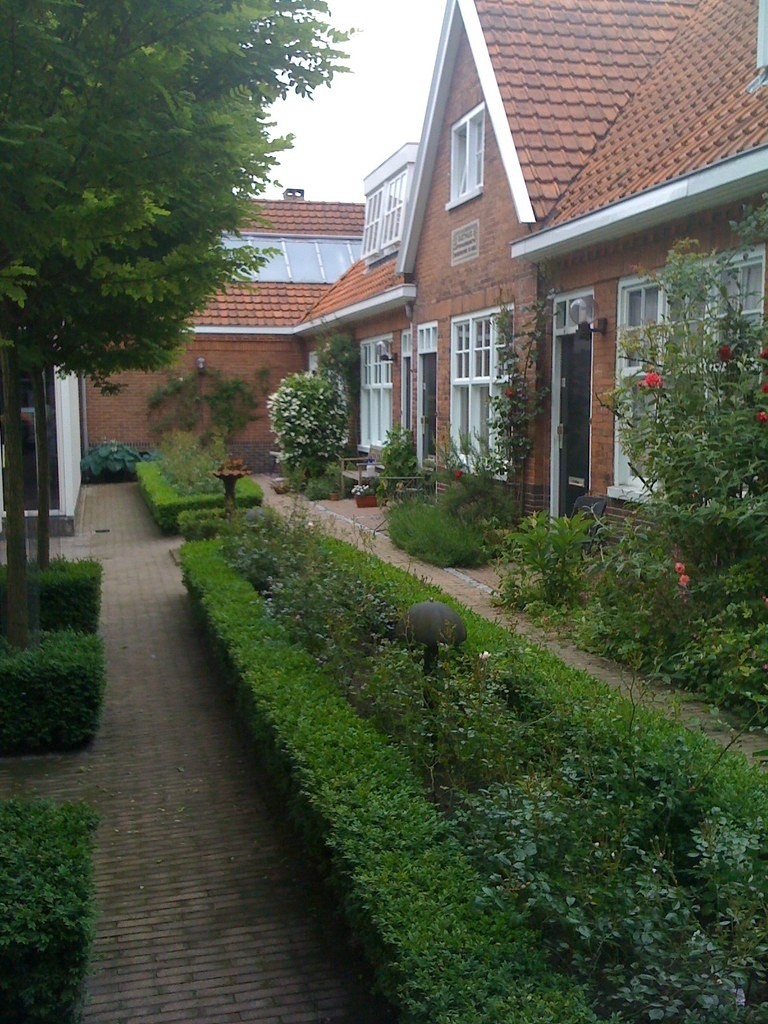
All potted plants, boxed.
[81,439,143,483]
[325,462,341,501]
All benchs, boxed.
[338,444,386,501]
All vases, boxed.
[356,496,377,508]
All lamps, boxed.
[569,295,608,341]
[196,356,208,374]
[375,339,398,366]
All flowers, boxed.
[350,484,375,496]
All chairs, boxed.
[556,495,608,565]
